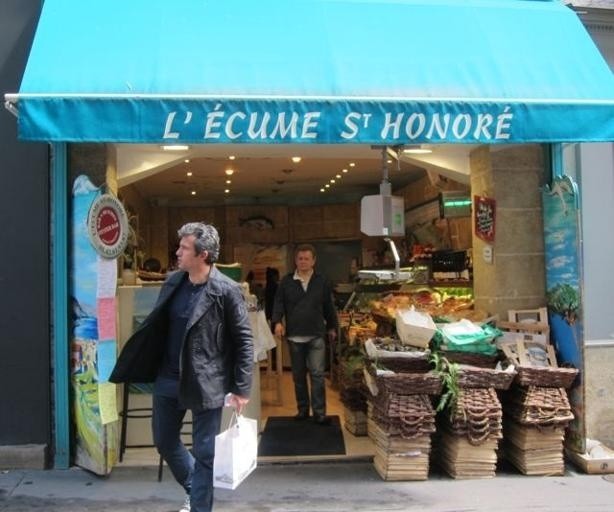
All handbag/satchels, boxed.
[211,407,258,491]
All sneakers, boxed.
[179,494,191,512]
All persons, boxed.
[264,266,279,335]
[272,243,337,423]
[108,222,254,512]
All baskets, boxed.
[446,387,504,446]
[431,249,466,272]
[509,386,575,434]
[518,365,580,389]
[448,363,518,393]
[444,349,499,370]
[375,372,444,395]
[362,355,435,372]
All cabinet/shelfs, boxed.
[226,205,288,244]
[294,203,356,239]
[118,282,262,451]
[331,280,473,413]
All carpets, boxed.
[258,415,346,457]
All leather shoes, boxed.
[312,415,332,425]
[295,412,308,420]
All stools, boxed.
[259,331,283,406]
[119,381,194,482]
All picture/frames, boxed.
[474,195,496,244]
